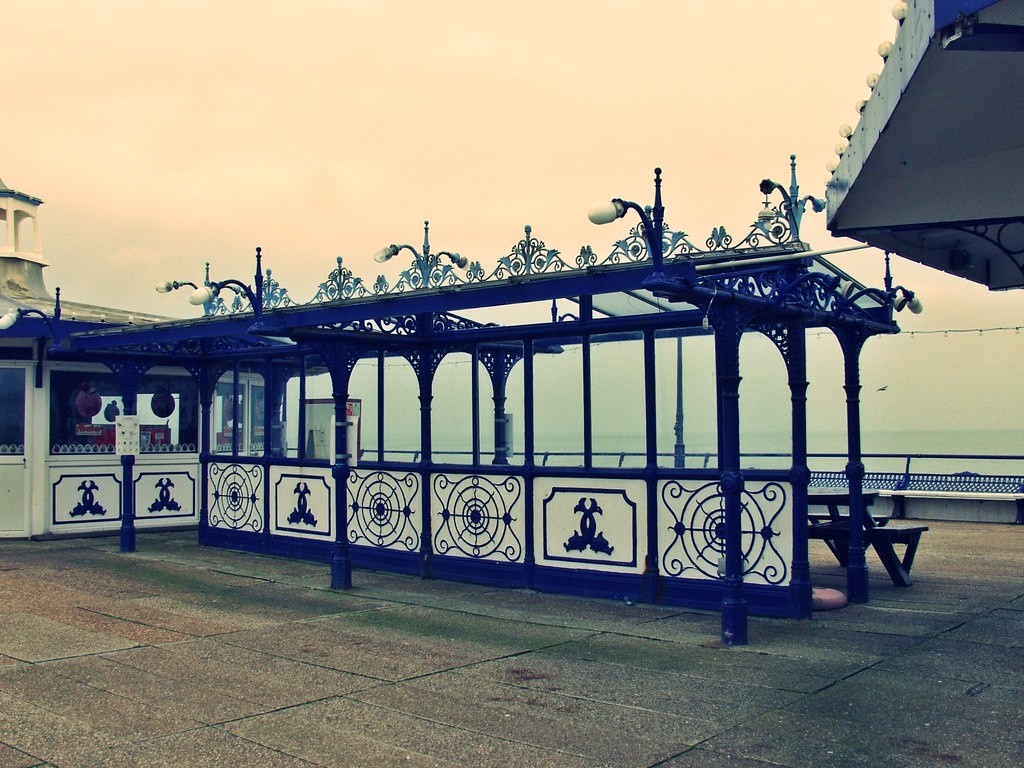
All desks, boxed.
[809,488,912,587]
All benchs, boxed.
[878,490,1024,525]
[807,514,929,572]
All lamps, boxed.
[588,168,667,278]
[373,219,471,286]
[758,154,826,239]
[0,287,61,344]
[156,262,210,315]
[189,246,265,325]
[776,251,922,320]
